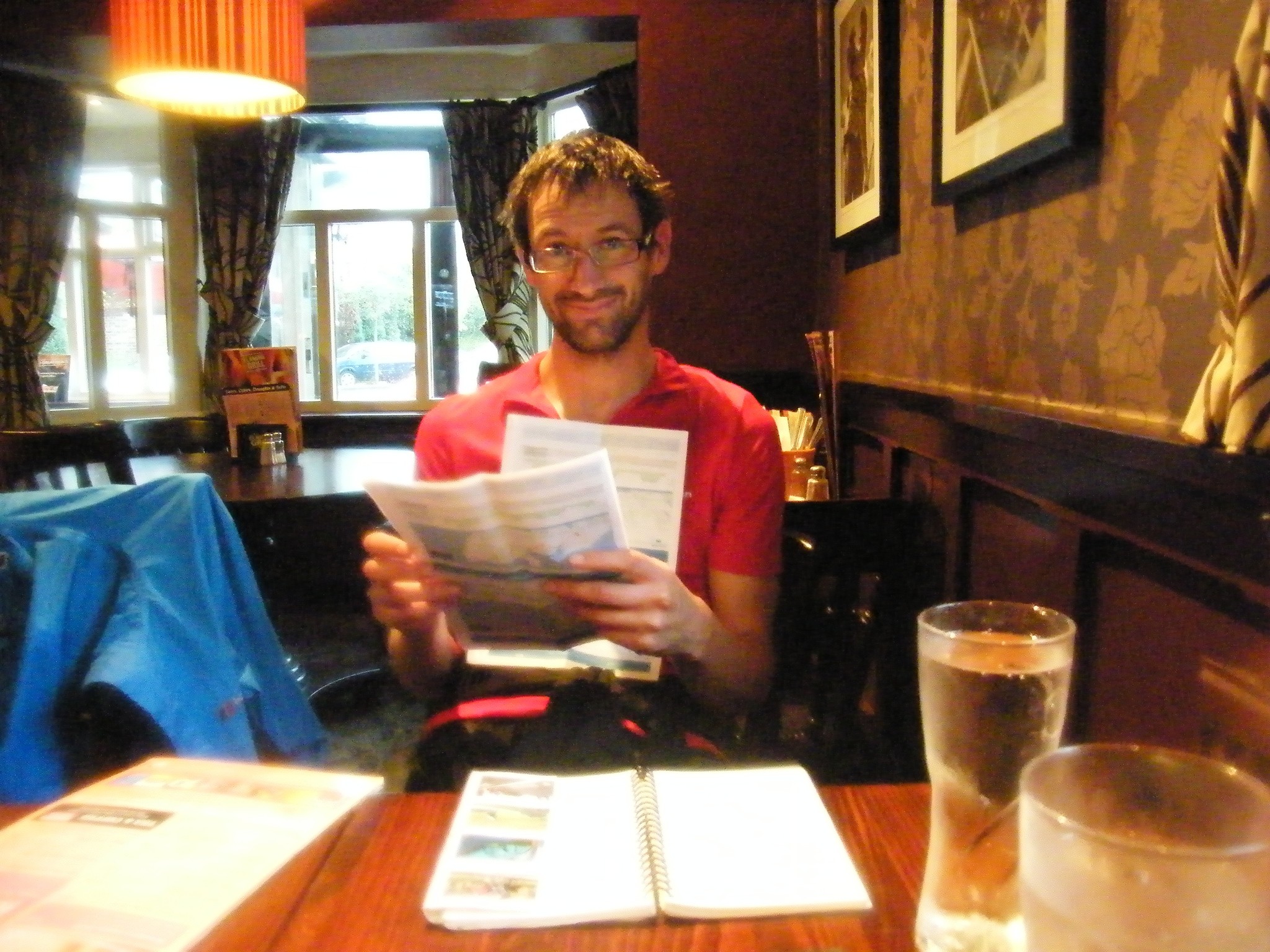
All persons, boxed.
[363,133,783,793]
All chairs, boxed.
[1,413,234,493]
[302,499,949,782]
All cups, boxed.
[1017,740,1270,951]
[913,599,1077,951]
[782,448,815,501]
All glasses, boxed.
[526,229,654,274]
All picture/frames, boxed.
[829,0,902,252]
[932,0,1108,205]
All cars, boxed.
[336,339,417,389]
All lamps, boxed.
[106,0,307,121]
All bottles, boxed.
[790,457,810,501]
[260,433,278,467]
[806,465,830,502]
[272,432,287,465]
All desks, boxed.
[1,753,931,951]
[14,444,419,611]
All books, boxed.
[421,759,873,929]
